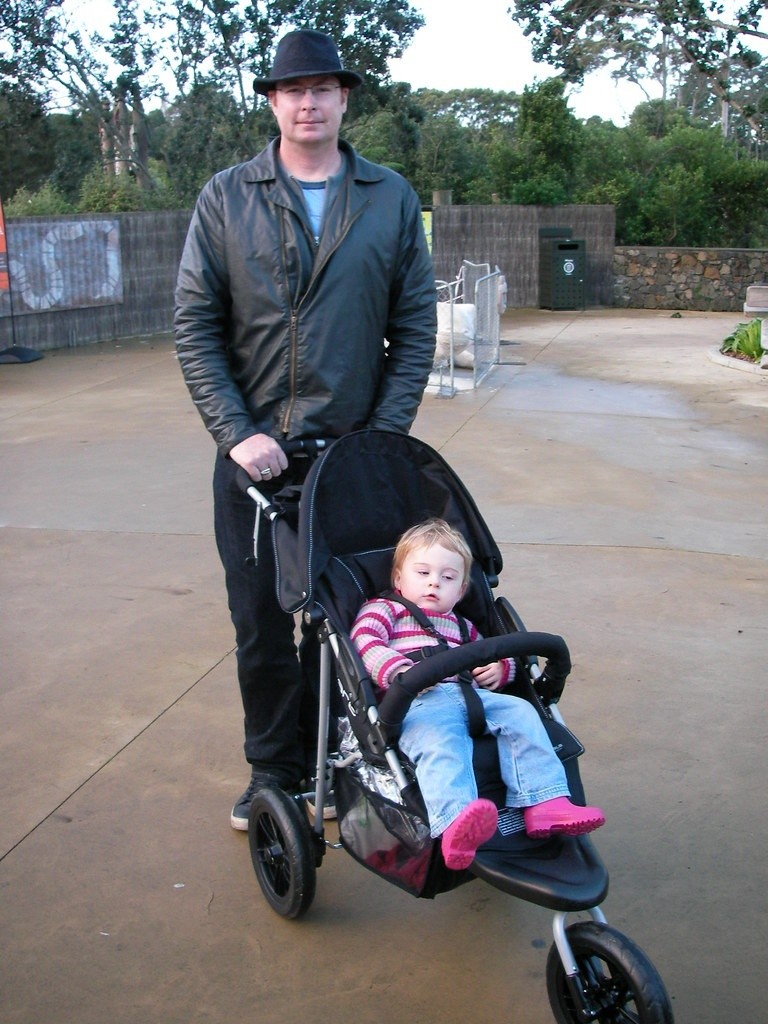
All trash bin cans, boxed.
[538,227,587,310]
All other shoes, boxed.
[524,797,605,839]
[440,799,498,869]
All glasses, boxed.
[274,83,341,100]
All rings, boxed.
[261,468,270,475]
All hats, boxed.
[253,30,363,97]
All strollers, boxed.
[234,425,685,1024]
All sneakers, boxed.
[306,788,338,820]
[230,778,302,831]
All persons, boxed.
[349,517,605,870]
[173,30,437,831]
